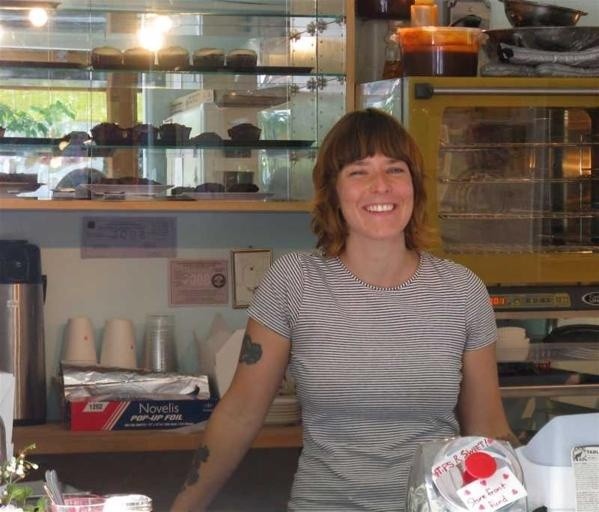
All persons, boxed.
[169,106,527,511]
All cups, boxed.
[141,314,178,371]
[62,317,97,364]
[99,317,137,368]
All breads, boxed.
[1,169,38,183]
[89,45,257,71]
[64,123,261,146]
[55,168,260,195]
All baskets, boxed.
[49,492,153,512]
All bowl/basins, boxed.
[497,0,588,27]
[480,26,599,52]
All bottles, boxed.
[403,436,527,512]
[383,20,404,78]
[411,0,438,25]
[1,238,48,426]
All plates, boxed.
[496,326,529,363]
[79,183,175,195]
[0,182,43,190]
[182,190,275,201]
[266,398,300,424]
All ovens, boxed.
[353,77,598,311]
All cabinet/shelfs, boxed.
[1,1,357,212]
